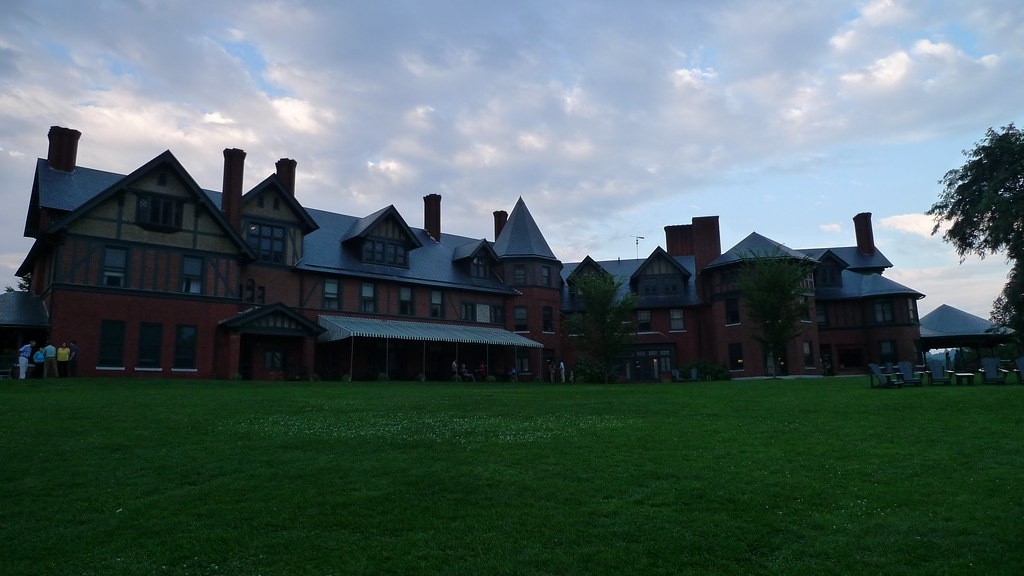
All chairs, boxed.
[978,358,1008,384]
[870,364,894,388]
[689,369,697,380]
[952,358,975,385]
[671,369,682,382]
[925,359,952,385]
[896,361,925,387]
[1012,358,1024,383]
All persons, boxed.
[448,358,487,383]
[17,341,80,380]
[508,359,565,384]
[945,350,961,372]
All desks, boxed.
[518,373,532,381]
